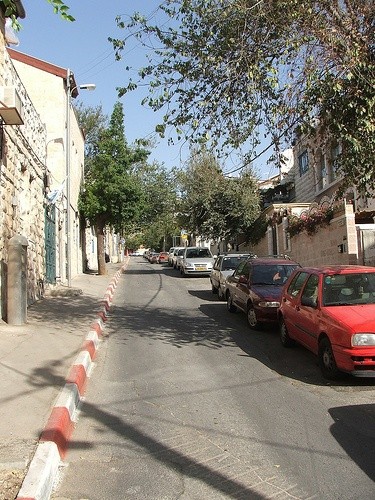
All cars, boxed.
[143,251,159,264]
[210,254,258,302]
[157,252,169,263]
[167,247,185,270]
[278,264,375,383]
[225,255,304,330]
[179,248,217,277]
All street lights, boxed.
[65,68,97,289]
